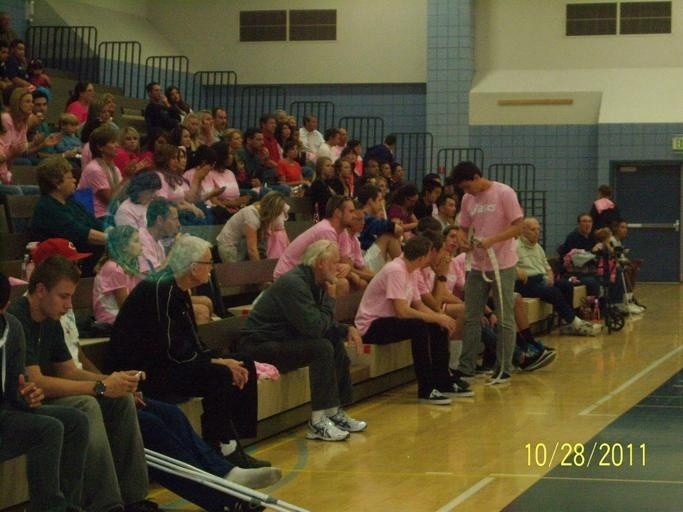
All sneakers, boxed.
[480,364,495,374]
[417,384,451,405]
[223,444,271,469]
[519,348,547,370]
[577,319,603,336]
[304,414,350,441]
[486,370,511,383]
[528,351,556,371]
[436,380,473,397]
[448,367,474,380]
[326,407,367,432]
[559,321,576,335]
[475,364,485,378]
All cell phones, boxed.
[131,370,142,382]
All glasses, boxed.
[192,257,214,265]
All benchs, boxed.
[180,220,322,297]
[0,316,312,511]
[0,230,86,286]
[333,268,589,380]
[9,277,226,371]
[210,251,371,329]
[0,66,329,232]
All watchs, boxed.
[436,275,448,282]
[93,380,107,399]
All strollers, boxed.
[545,245,624,334]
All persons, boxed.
[141,128,171,169]
[165,86,194,125]
[80,101,109,144]
[341,147,360,199]
[212,107,229,137]
[273,109,287,124]
[181,113,203,151]
[390,162,404,194]
[31,90,57,153]
[242,127,292,196]
[368,176,389,221]
[112,127,153,177]
[93,225,146,327]
[109,236,271,469]
[422,232,450,313]
[114,171,162,231]
[287,116,298,129]
[0,88,61,186]
[443,176,463,199]
[77,127,151,225]
[354,236,475,404]
[593,227,615,254]
[363,134,396,167]
[273,195,356,296]
[424,174,441,185]
[335,159,351,202]
[1,12,17,44]
[434,193,456,229]
[358,159,380,190]
[443,225,460,257]
[347,139,364,176]
[144,82,183,130]
[258,114,281,165]
[305,156,344,220]
[413,180,442,221]
[6,256,163,512]
[299,113,325,153]
[221,129,273,200]
[515,218,604,336]
[0,274,90,512]
[236,240,367,441]
[559,215,644,314]
[211,191,287,263]
[172,125,195,171]
[0,42,12,88]
[290,126,303,142]
[99,94,118,125]
[318,128,338,164]
[197,109,218,146]
[211,141,253,213]
[357,184,403,273]
[380,161,392,179]
[265,202,289,259]
[590,185,620,229]
[339,205,374,281]
[176,147,187,174]
[154,143,190,198]
[386,185,420,236]
[22,239,282,512]
[183,144,232,224]
[275,122,293,146]
[31,58,52,102]
[8,39,31,87]
[64,80,95,124]
[449,161,524,384]
[335,127,348,158]
[138,198,181,274]
[31,155,108,278]
[610,220,628,247]
[57,113,81,152]
[276,138,312,188]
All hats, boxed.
[32,238,93,265]
[325,194,347,217]
